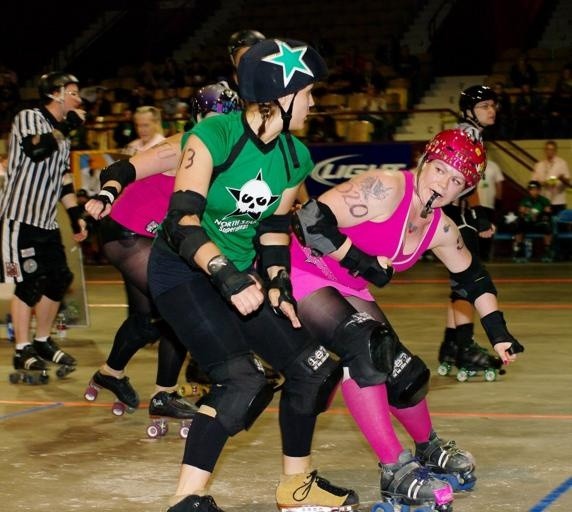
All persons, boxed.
[438,86,507,373]
[69,80,243,422]
[1,71,88,370]
[0,56,572,264]
[146,39,357,512]
[185,29,283,387]
[252,129,525,506]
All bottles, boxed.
[6,309,37,342]
[57,312,66,338]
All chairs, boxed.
[492,212,519,262]
[289,47,572,141]
[550,207,572,262]
[523,216,554,262]
[0,65,216,160]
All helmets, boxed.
[38,72,79,96]
